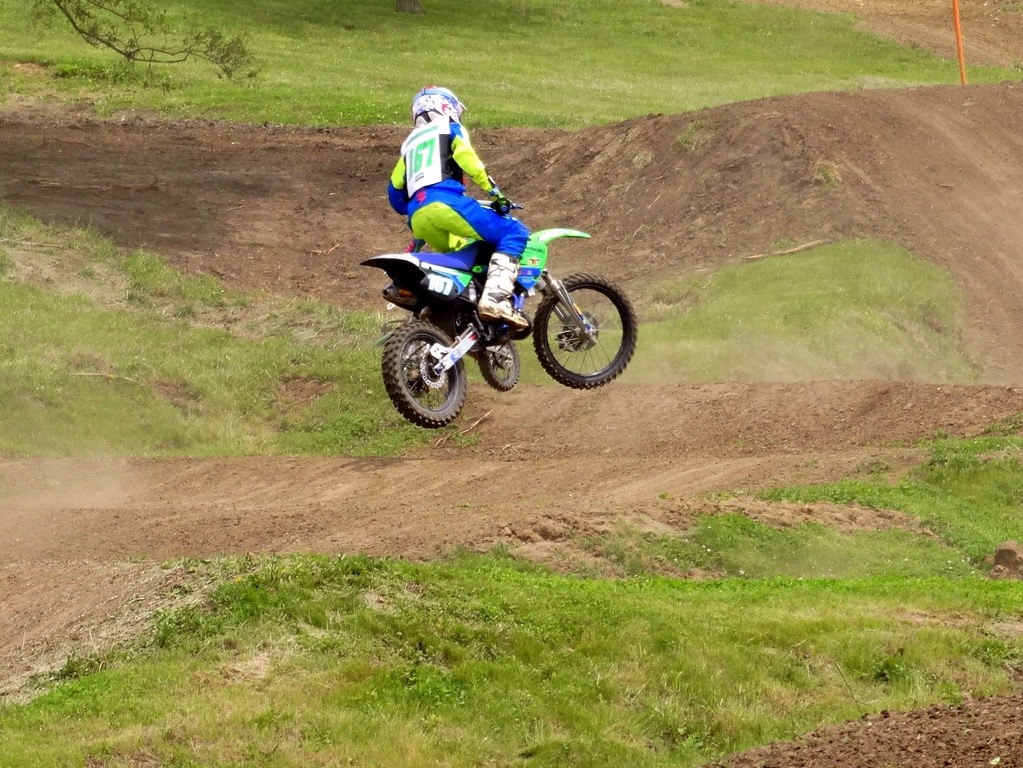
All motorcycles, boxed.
[359,200,638,429]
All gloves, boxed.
[491,199,510,214]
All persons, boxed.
[387,85,530,329]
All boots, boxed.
[477,253,530,327]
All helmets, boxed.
[411,85,469,124]
